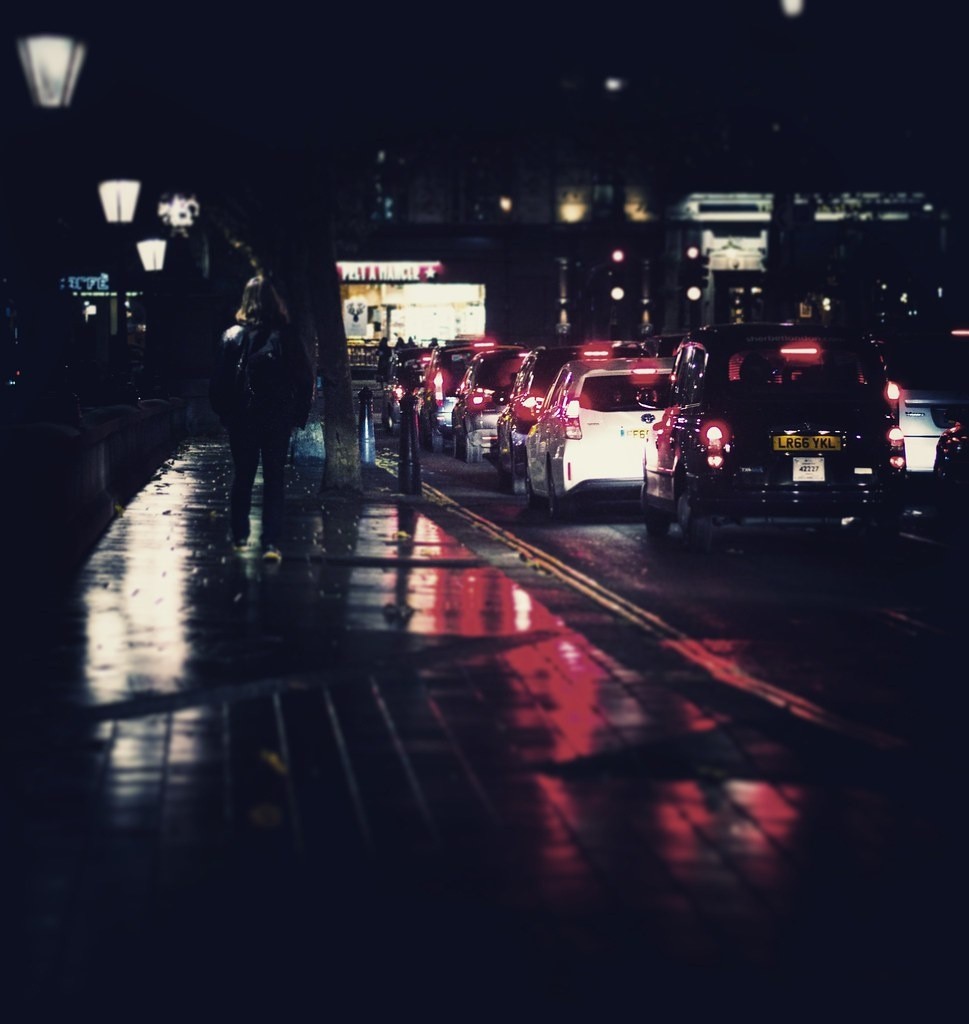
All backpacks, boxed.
[234,315,314,424]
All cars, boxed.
[499,342,656,496]
[446,348,531,463]
[636,321,907,551]
[419,345,494,454]
[522,357,674,523]
[890,326,969,496]
[379,347,432,431]
[928,405,968,545]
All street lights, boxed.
[98,168,143,404]
[16,25,87,417]
[135,231,169,395]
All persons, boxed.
[376,338,437,383]
[209,272,314,560]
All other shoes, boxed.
[262,544,281,561]
[231,538,247,552]
